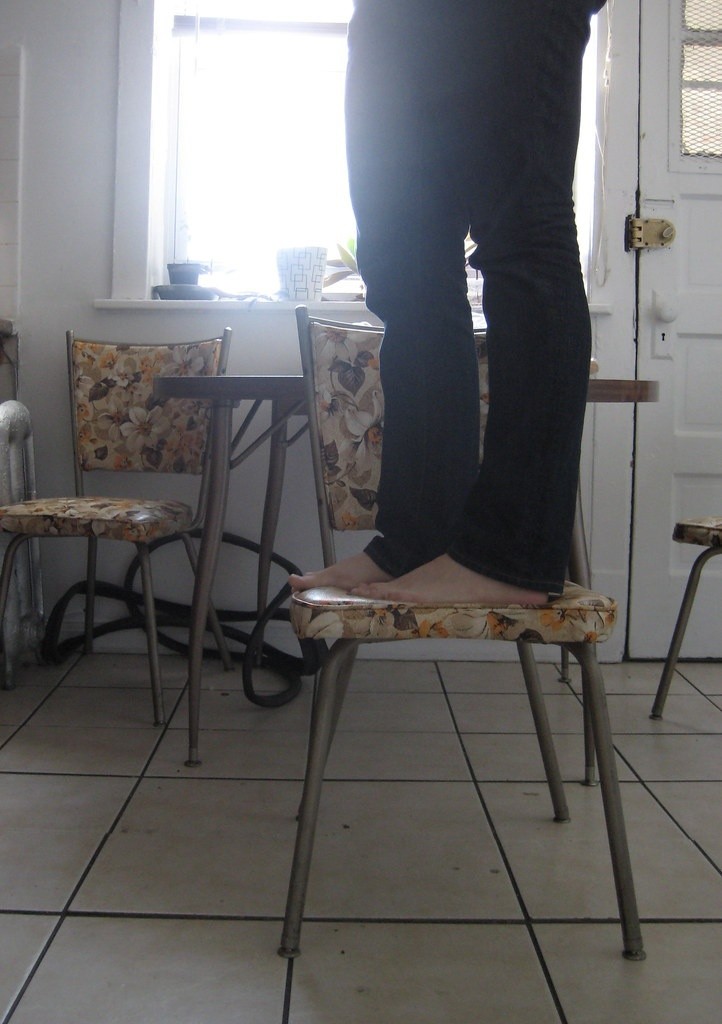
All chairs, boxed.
[648,513,722,721]
[276,305,646,963]
[0,325,234,728]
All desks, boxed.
[151,375,662,788]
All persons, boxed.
[289,0,607,601]
